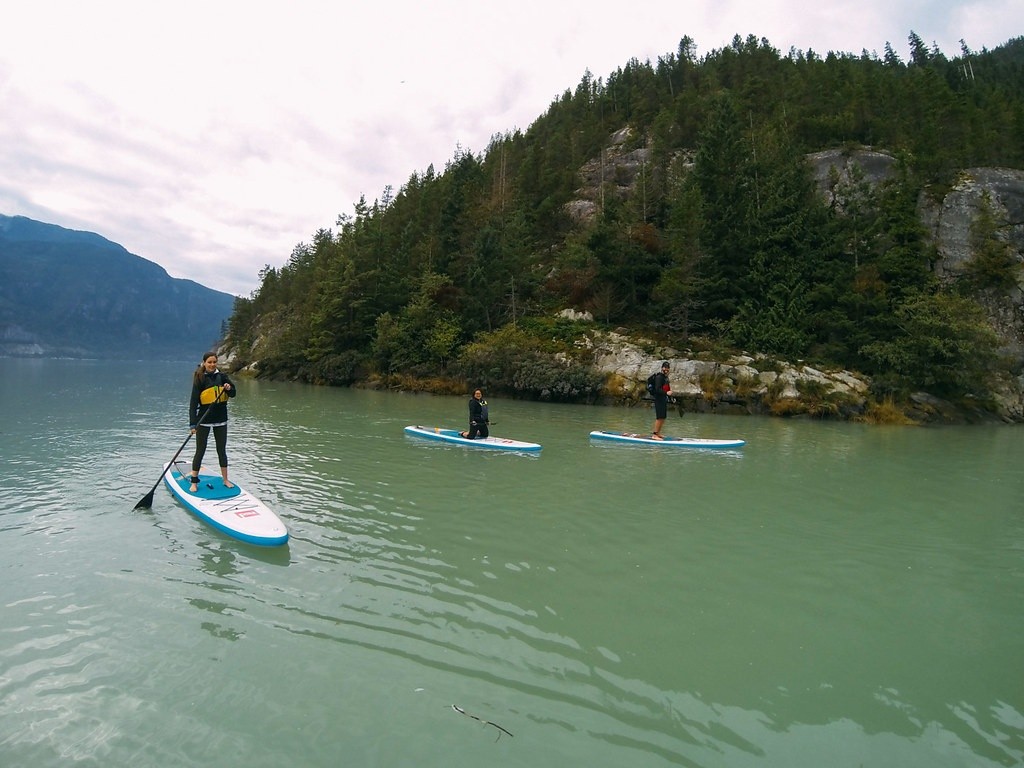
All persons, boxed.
[652,361,673,441]
[189,352,236,492]
[458,388,491,440]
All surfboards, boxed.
[403,426,541,453]
[588,430,746,449]
[163,459,291,549]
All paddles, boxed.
[667,394,685,418]
[470,422,499,426]
[131,382,229,510]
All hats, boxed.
[662,361,671,368]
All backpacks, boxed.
[646,373,657,395]
